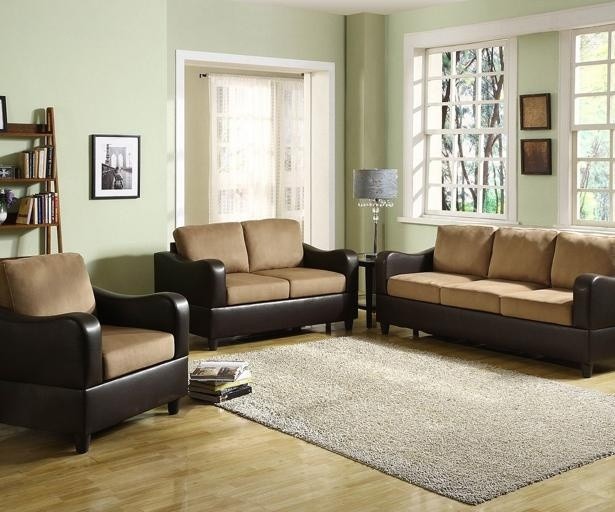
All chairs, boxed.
[0,251,192,453]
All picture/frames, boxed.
[90,133,141,200]
[520,93,552,131]
[0,95,9,130]
[520,138,553,176]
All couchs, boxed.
[376,223,615,380]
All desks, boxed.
[358,251,379,331]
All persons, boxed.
[113,166,124,189]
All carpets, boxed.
[188,335,614,506]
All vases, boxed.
[0,202,8,226]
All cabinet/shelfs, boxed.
[0,107,62,263]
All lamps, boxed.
[350,168,400,259]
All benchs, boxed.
[154,218,359,352]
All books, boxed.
[16,191,58,225]
[187,361,252,403]
[19,144,52,179]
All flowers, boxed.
[0,186,19,211]
